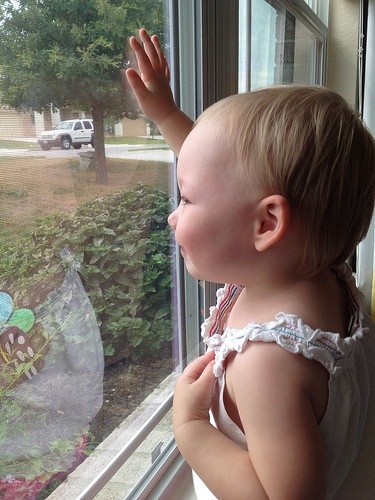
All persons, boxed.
[126,27,375,500]
[0,31,151,500]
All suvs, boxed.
[38,118,95,150]
